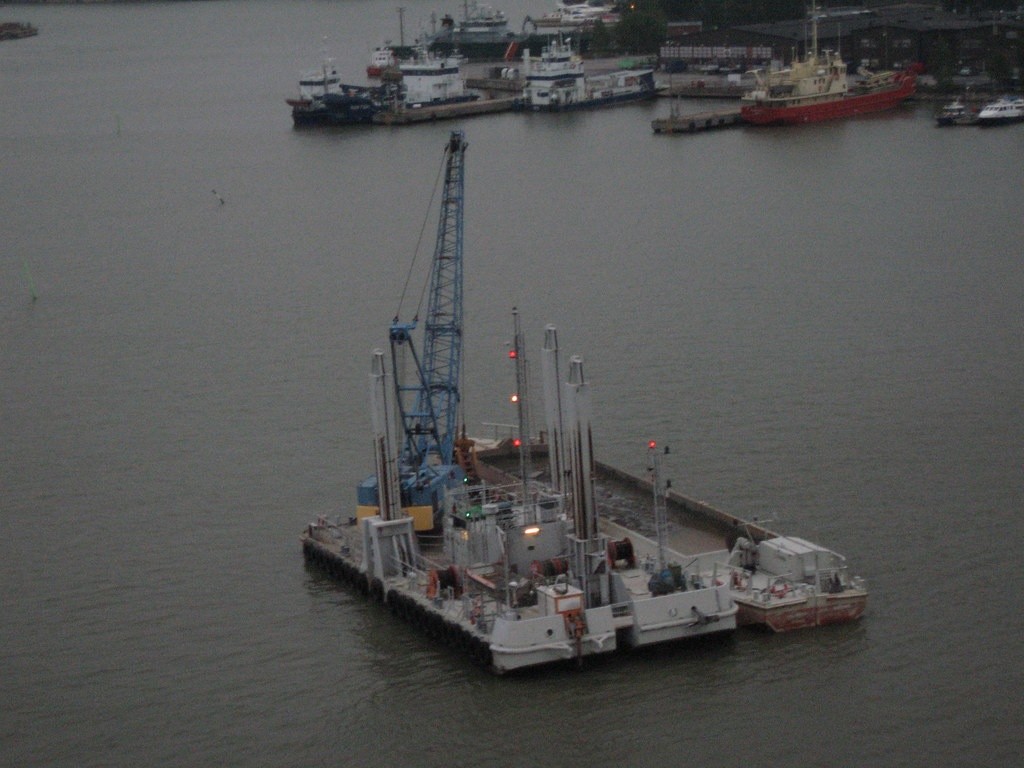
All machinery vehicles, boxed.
[357,128,472,534]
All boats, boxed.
[284,36,374,130]
[367,0,621,79]
[515,31,669,113]
[978,96,1024,118]
[299,306,873,676]
[0,21,38,41]
[741,0,915,128]
[935,101,965,119]
